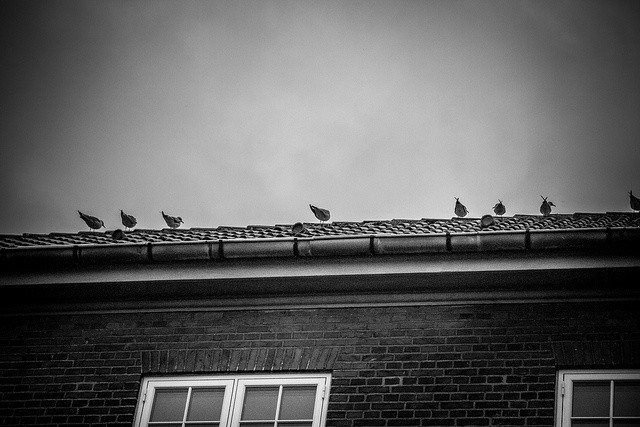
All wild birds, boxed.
[78,210,106,231]
[120,210,138,231]
[539,194,556,217]
[308,204,330,223]
[494,199,506,216]
[161,211,184,228]
[627,190,640,211]
[453,197,469,217]
